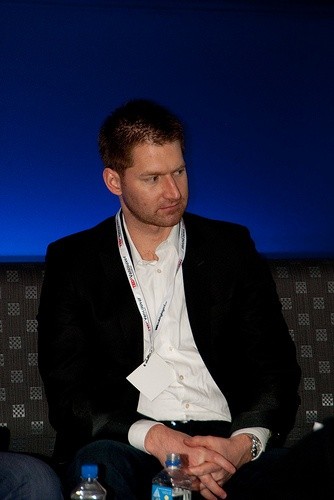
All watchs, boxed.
[243,433,262,458]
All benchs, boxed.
[0,259,334,457]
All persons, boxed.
[0,451,65,500]
[37,98,302,500]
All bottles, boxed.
[151,453,192,500]
[70,464,106,500]
